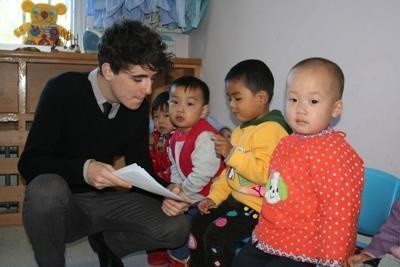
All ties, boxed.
[103,102,112,120]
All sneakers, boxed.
[147,249,190,267]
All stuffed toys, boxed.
[13,0,81,54]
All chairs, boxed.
[355,166,399,248]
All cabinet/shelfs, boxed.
[0,51,201,227]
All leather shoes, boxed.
[88,232,124,267]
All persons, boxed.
[18,21,192,267]
[342,197,400,267]
